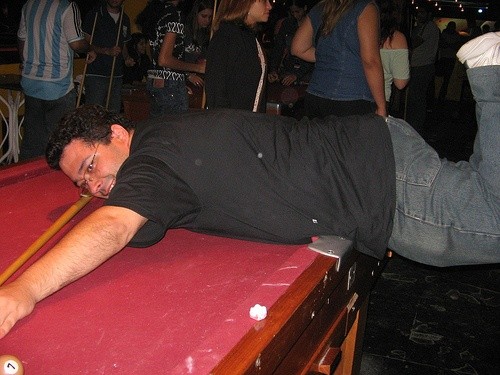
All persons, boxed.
[17,0,96,162]
[81,0,500,144]
[0,33,500,341]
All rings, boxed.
[197,82,200,85]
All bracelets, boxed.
[186,73,192,79]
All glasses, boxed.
[79,137,106,198]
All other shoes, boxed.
[456,32,500,68]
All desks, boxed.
[0,158,390,375]
[0,73,25,165]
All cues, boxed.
[76,12,98,108]
[201,0,217,109]
[105,2,125,110]
[0,193,93,287]
[404,16,414,120]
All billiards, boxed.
[0,355,24,375]
